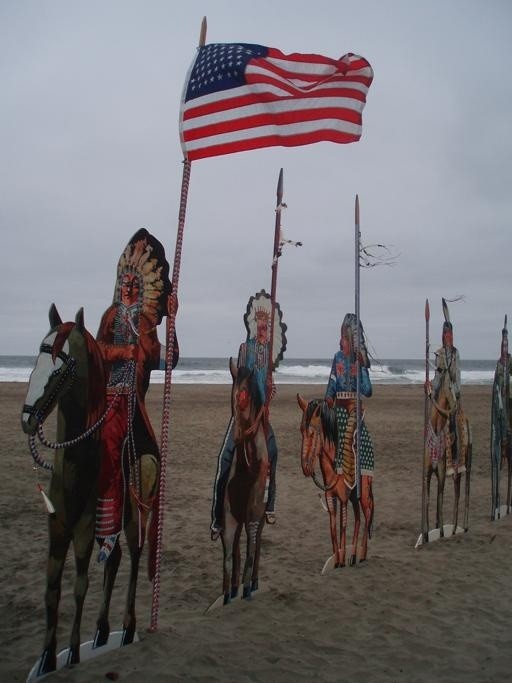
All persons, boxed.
[210,290,294,541]
[324,311,371,505]
[434,295,463,480]
[94,227,174,563]
[495,314,512,399]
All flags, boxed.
[178,43,374,162]
